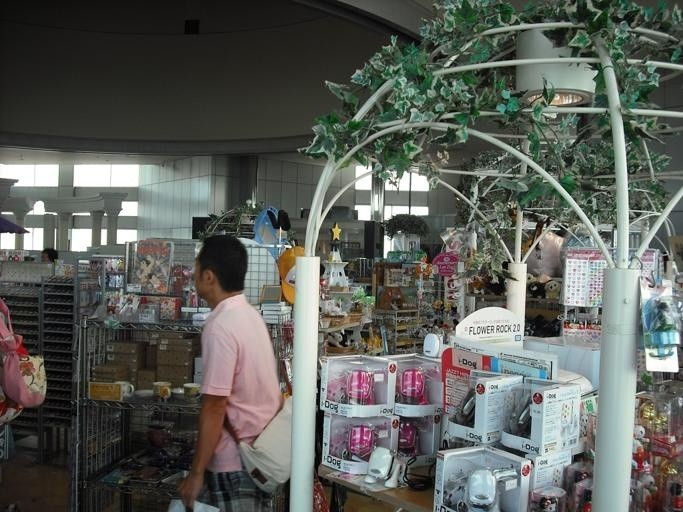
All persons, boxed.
[536,468,683,512]
[39,244,61,264]
[175,233,280,512]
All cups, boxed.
[115,380,201,404]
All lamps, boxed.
[240,214,256,225]
[516,28,599,107]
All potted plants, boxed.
[381,213,430,251]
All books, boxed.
[86,240,292,329]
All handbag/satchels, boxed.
[238,396,292,494]
[0,298,47,424]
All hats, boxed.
[279,246,303,303]
